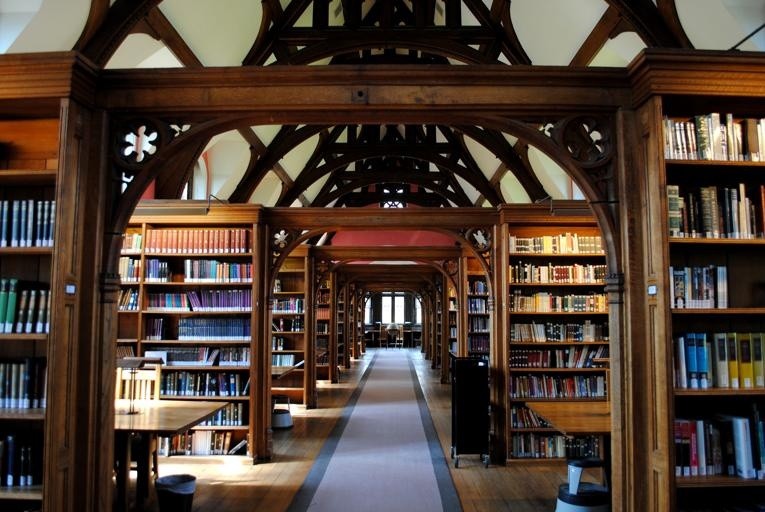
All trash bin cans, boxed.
[554,459,612,512]
[271,395,294,429]
[155,474,196,512]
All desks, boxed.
[112,398,230,511]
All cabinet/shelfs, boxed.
[633,97,765,512]
[1,93,60,512]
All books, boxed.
[438,302,440,367]
[662,111,764,484]
[508,235,610,460]
[117,228,252,457]
[349,298,355,358]
[338,294,344,365]
[0,199,56,491]
[449,298,457,382]
[272,278,304,368]
[316,279,330,365]
[468,280,489,359]
